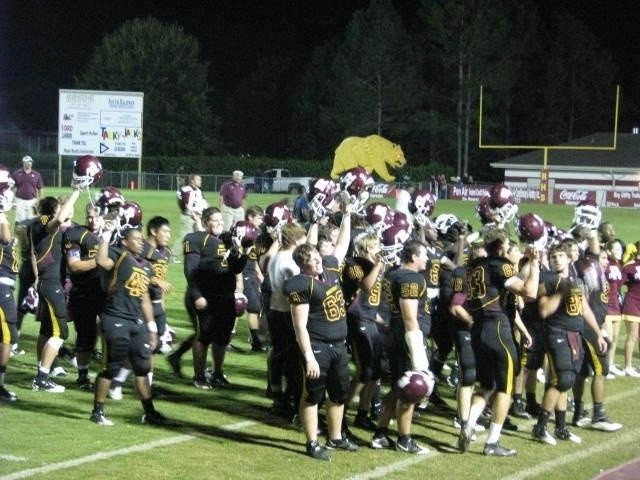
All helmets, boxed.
[233,168,457,266]
[157,324,176,354]
[71,155,142,230]
[22,286,40,314]
[0,165,15,213]
[476,185,602,252]
[234,293,248,317]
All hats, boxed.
[23,155,33,163]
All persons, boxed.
[0,186,180,427]
[164,167,640,461]
[10,156,43,236]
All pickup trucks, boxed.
[238,168,318,195]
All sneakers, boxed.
[251,340,431,460]
[0,343,244,426]
[170,256,182,264]
[447,365,640,454]
